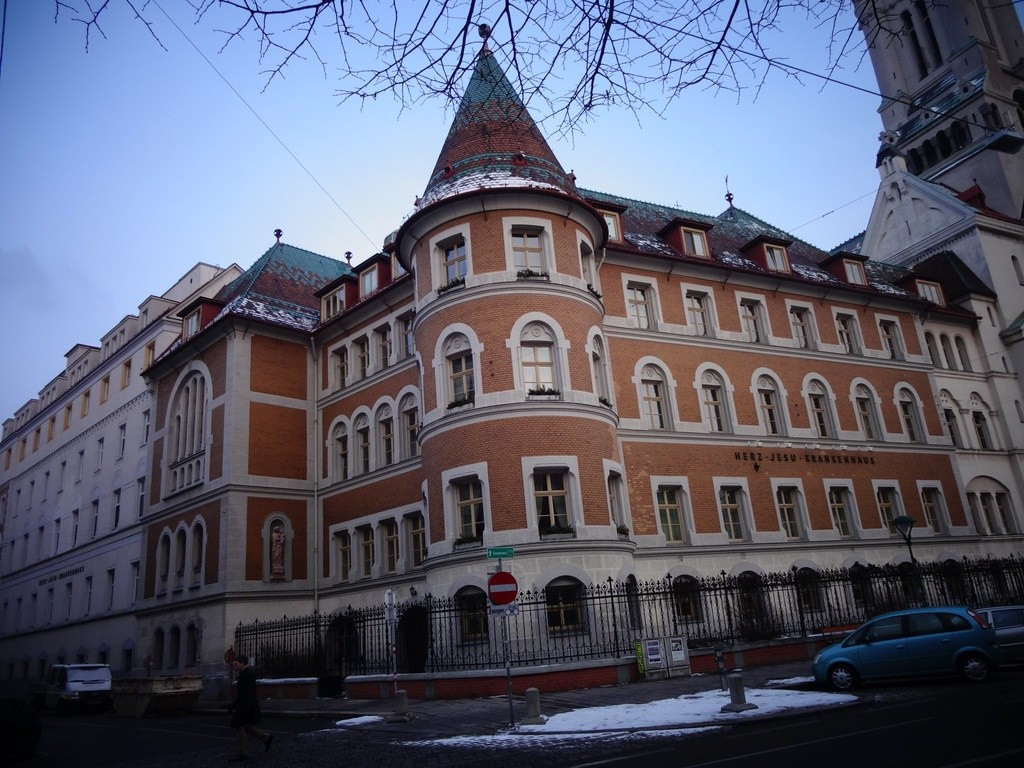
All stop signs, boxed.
[488,571,518,606]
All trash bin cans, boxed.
[634,635,692,681]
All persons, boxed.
[228,654,273,761]
[271,527,285,566]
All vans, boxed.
[31,663,113,712]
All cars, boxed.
[953,605,1024,668]
[810,606,1001,692]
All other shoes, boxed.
[264,733,273,755]
[229,754,249,762]
